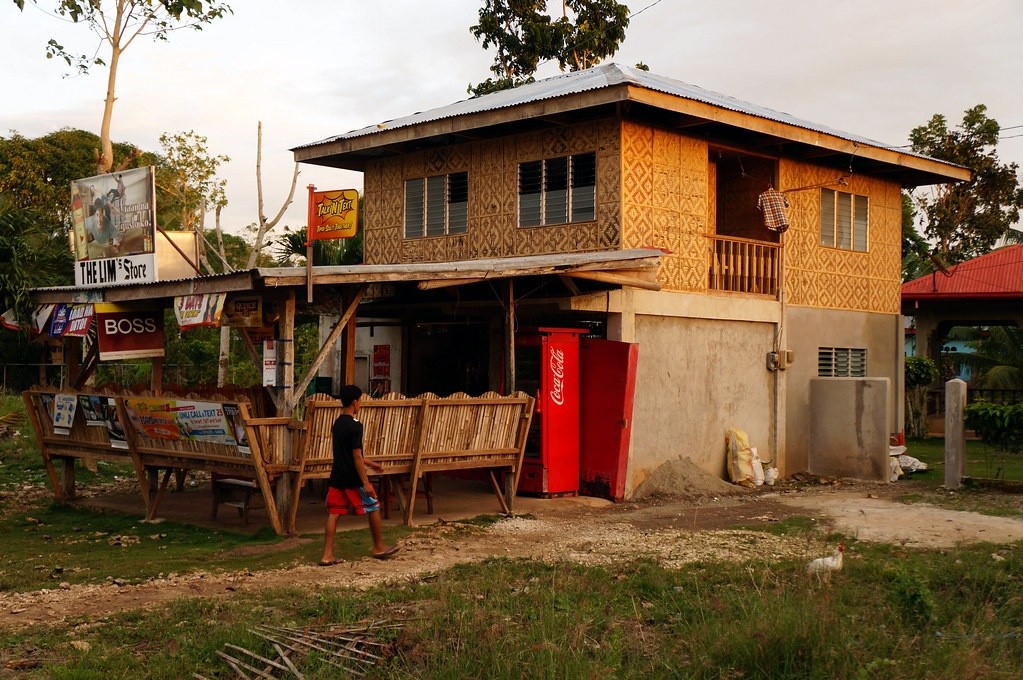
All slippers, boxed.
[319,559,343,566]
[374,545,403,559]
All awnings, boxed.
[19,247,663,529]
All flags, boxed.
[313,189,358,239]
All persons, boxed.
[319,386,402,566]
[85,198,120,244]
[105,174,124,204]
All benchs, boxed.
[21,385,535,537]
